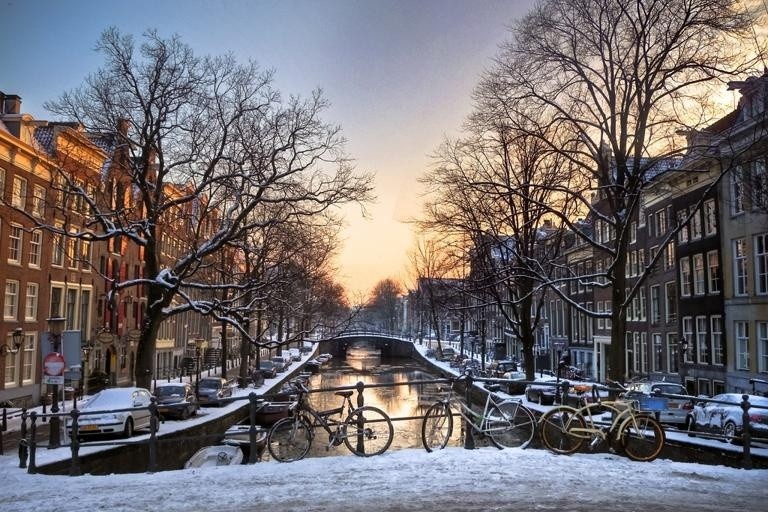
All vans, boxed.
[612,381,691,430]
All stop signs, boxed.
[43,351,64,377]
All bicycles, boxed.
[262,381,394,464]
[537,380,666,461]
[418,367,535,456]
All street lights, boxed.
[675,336,687,387]
[44,311,66,448]
[192,334,205,386]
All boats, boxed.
[182,444,244,468]
[255,353,333,428]
[220,424,267,454]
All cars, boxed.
[684,393,767,447]
[426,341,609,415]
[66,386,160,443]
[233,332,320,388]
[194,376,234,409]
[152,382,197,422]
